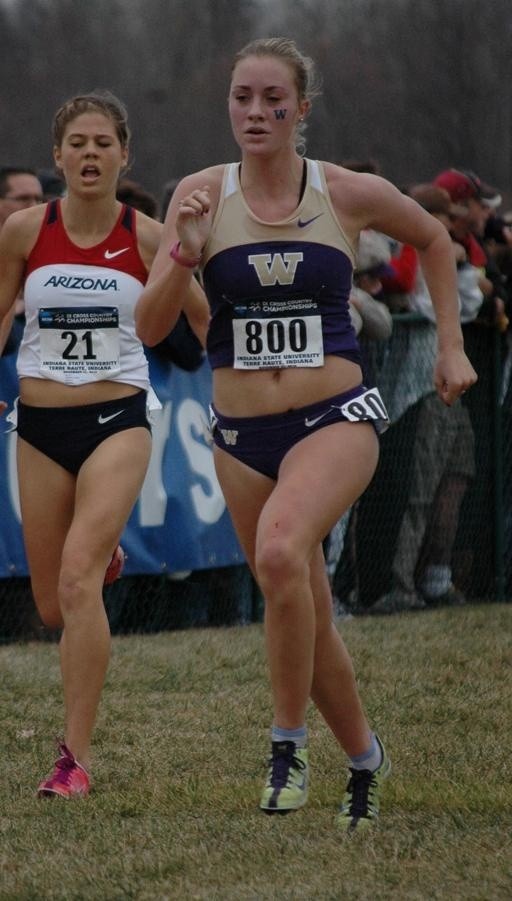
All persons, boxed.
[0,90,213,801]
[0,161,512,626]
[136,38,478,840]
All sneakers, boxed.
[334,735,392,831]
[38,744,90,799]
[260,740,308,809]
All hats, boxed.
[434,168,502,208]
